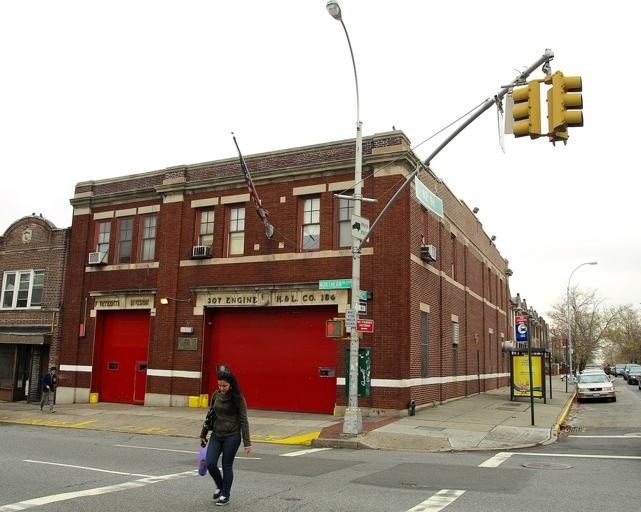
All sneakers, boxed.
[217,495,230,505]
[212,487,221,503]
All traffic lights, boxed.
[553,70,584,131]
[511,79,542,140]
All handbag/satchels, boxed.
[203,406,217,430]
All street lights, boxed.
[325,0,363,435]
[567,261,598,384]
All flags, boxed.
[230,131,272,226]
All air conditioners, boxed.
[193,245,212,258]
[89,251,109,264]
[421,245,436,262]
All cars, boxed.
[576,363,641,404]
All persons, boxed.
[40,367,59,413]
[200,370,254,506]
[605,364,612,380]
